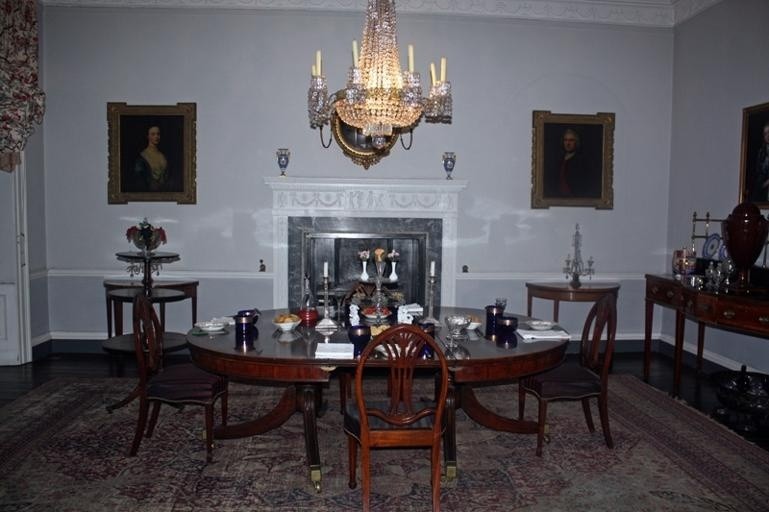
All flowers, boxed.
[358,250,370,261]
[388,249,400,262]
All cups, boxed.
[232,334,256,351]
[347,325,371,345]
[445,340,472,361]
[415,322,435,342]
[496,316,519,333]
[483,324,496,342]
[231,315,255,334]
[485,305,504,324]
[444,313,473,339]
[418,342,435,361]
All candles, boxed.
[352,37,359,68]
[311,66,315,76]
[441,55,447,83]
[431,61,437,86]
[316,47,322,77]
[323,262,330,278]
[430,260,435,278]
[408,43,415,72]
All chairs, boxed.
[343,324,449,512]
[517,294,617,456]
[129,293,229,466]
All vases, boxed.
[360,262,369,281]
[389,262,398,282]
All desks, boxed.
[525,282,622,374]
[103,279,199,338]
[644,271,769,387]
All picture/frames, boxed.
[531,110,617,210]
[331,112,400,171]
[737,101,769,210]
[106,101,197,206]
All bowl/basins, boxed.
[272,320,301,331]
[271,326,305,343]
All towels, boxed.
[517,326,573,344]
[396,302,424,313]
[312,341,358,363]
[210,315,237,326]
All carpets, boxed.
[0,371,769,511]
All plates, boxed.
[718,243,729,262]
[526,321,558,330]
[496,333,518,350]
[702,233,721,259]
[466,329,485,341]
[195,322,229,332]
[466,321,483,330]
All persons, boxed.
[131,126,175,192]
[545,126,598,200]
